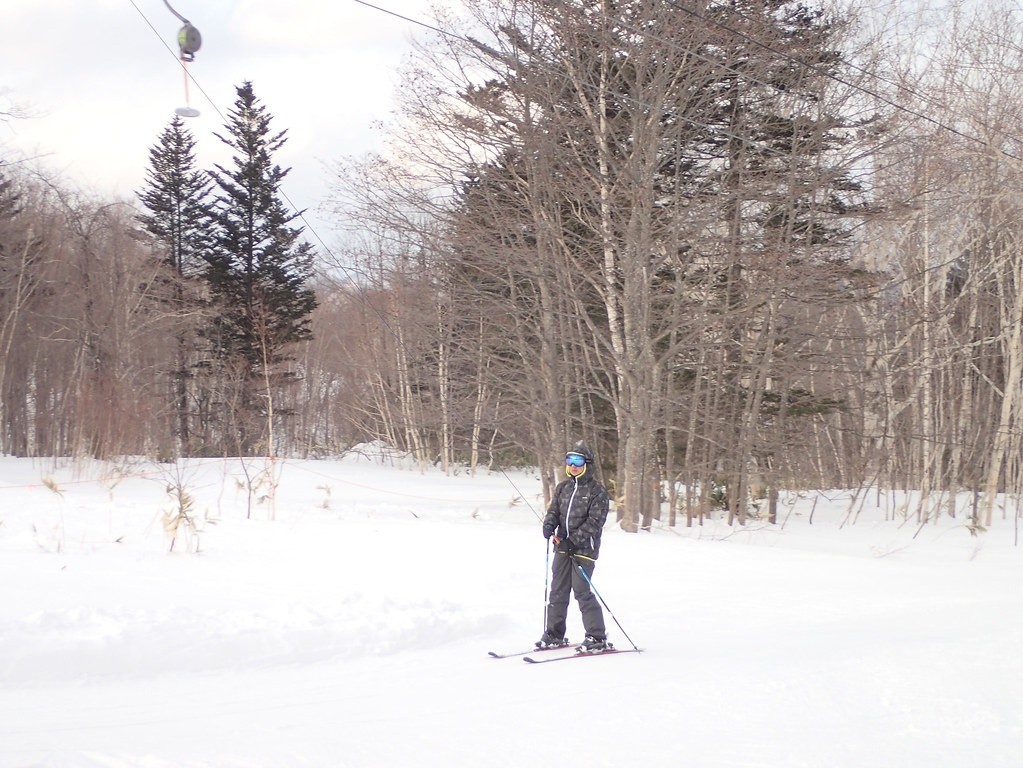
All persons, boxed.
[535,439,609,653]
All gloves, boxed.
[559,538,575,556]
[543,524,555,539]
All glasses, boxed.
[565,454,586,467]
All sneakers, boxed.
[535,631,564,650]
[574,635,607,655]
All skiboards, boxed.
[486,641,646,664]
